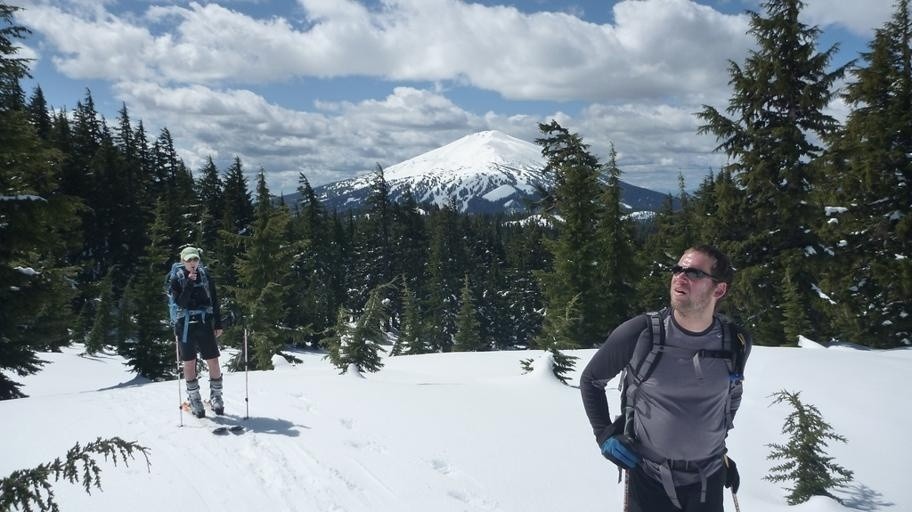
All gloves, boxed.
[725,456,739,493]
[601,433,642,468]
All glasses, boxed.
[672,266,721,282]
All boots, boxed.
[186,379,204,415]
[210,379,223,410]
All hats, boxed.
[181,247,200,261]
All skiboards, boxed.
[182,399,243,433]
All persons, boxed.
[167,247,225,417]
[580,245,752,512]
